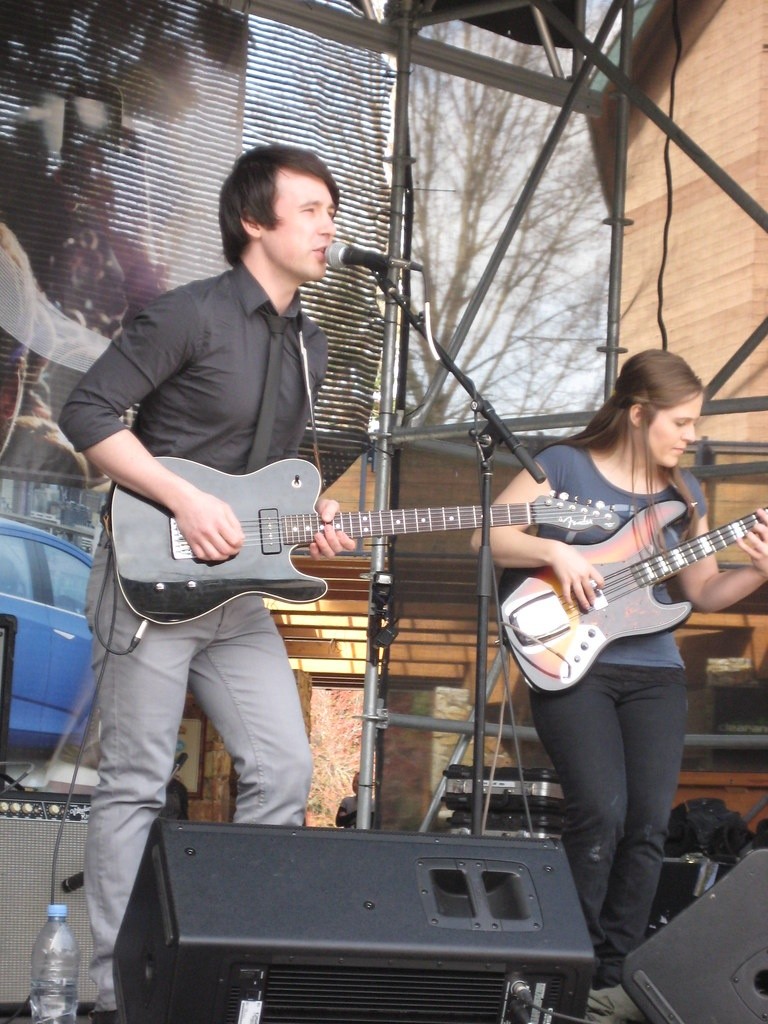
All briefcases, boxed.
[441,764,566,813]
[446,810,566,840]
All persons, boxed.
[472,349,768,991]
[58,145,356,1024]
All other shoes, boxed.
[587,984,645,1021]
[92,1010,119,1024]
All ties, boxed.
[244,301,293,475]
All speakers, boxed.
[0,790,99,1010]
[113,818,594,1024]
[620,849,768,1024]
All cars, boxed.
[0,516,100,748]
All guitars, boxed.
[105,455,621,628]
[503,499,768,695]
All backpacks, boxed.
[665,797,754,864]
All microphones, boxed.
[62,872,84,892]
[325,242,425,270]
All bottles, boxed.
[28,906,78,1024]
